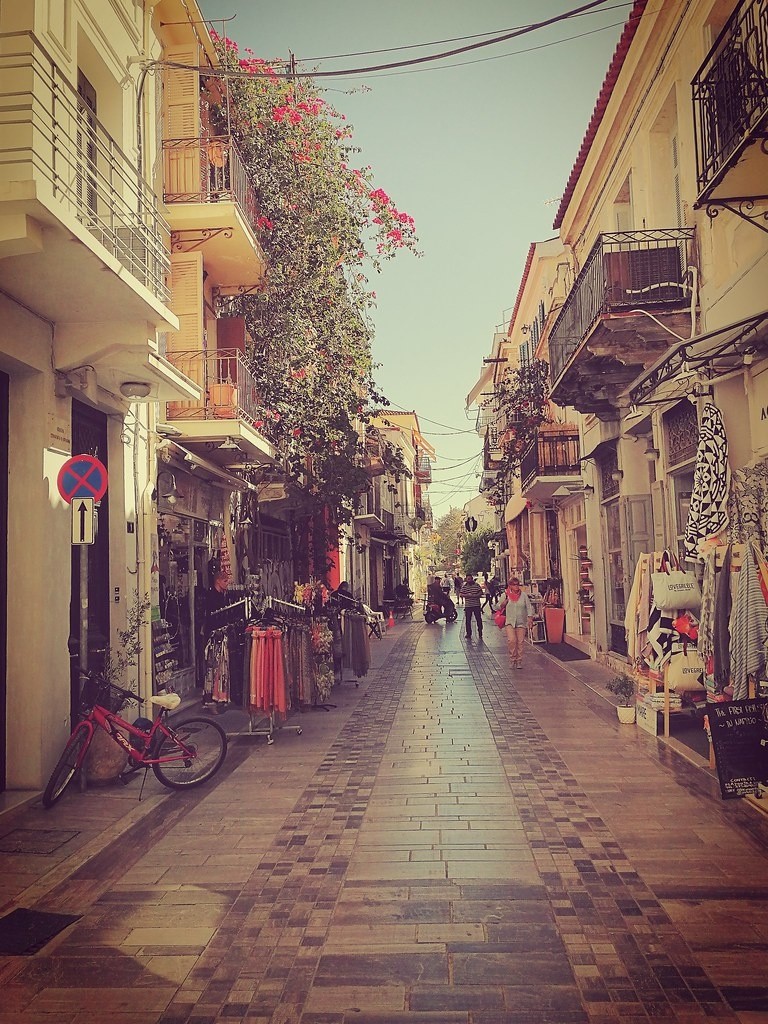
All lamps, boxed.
[644,449,660,461]
[218,437,241,453]
[521,324,529,334]
[624,405,643,421]
[672,361,696,382]
[231,504,252,544]
[551,485,582,496]
[152,470,184,506]
[120,384,150,399]
[583,484,594,496]
[495,495,506,514]
[611,470,622,482]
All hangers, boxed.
[321,591,368,617]
[210,603,305,629]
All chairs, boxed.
[363,604,385,634]
[396,592,414,619]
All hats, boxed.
[435,577,441,580]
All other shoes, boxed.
[479,629,482,637]
[446,618,454,623]
[507,661,517,669]
[516,661,522,668]
[465,632,472,638]
[456,602,459,604]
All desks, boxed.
[381,600,394,617]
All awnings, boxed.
[172,440,248,488]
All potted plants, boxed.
[580,571,588,578]
[73,588,151,786]
[605,673,635,724]
[579,557,592,569]
[582,613,590,623]
[579,545,588,558]
[580,579,594,590]
[576,589,589,602]
[578,594,595,612]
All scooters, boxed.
[423,589,458,624]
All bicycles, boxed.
[39,664,229,810]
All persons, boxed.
[430,577,454,623]
[426,572,500,613]
[397,579,414,606]
[496,577,533,669]
[202,572,230,690]
[328,581,355,608]
[460,574,483,638]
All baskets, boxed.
[80,680,125,724]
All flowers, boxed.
[209,28,420,590]
[475,359,554,506]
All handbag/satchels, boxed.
[669,636,706,692]
[651,550,702,611]
[671,610,701,640]
[494,593,508,630]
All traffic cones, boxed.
[387,610,396,628]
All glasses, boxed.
[510,583,519,587]
